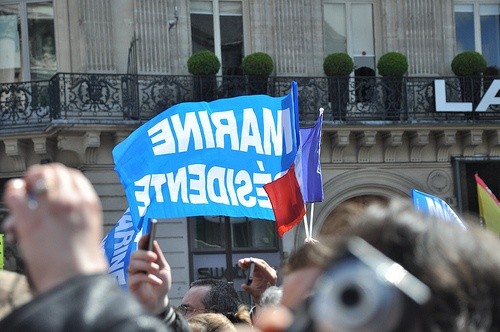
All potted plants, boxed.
[451,50,487,119]
[189,51,220,101]
[242,51,274,95]
[325,53,354,118]
[377,51,408,119]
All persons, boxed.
[0,163,500,332]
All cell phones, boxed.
[246,263,255,284]
[144,218,157,274]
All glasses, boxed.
[177,305,198,315]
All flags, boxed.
[102,207,151,289]
[112,81,300,232]
[262,112,325,238]
[474,174,500,238]
[411,188,467,229]
